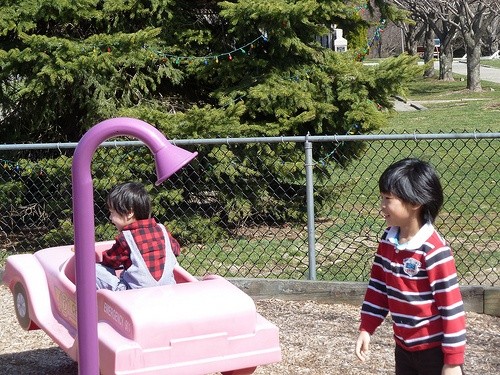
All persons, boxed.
[354,156,466,375]
[95,179,180,292]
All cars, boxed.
[6,239,283,375]
